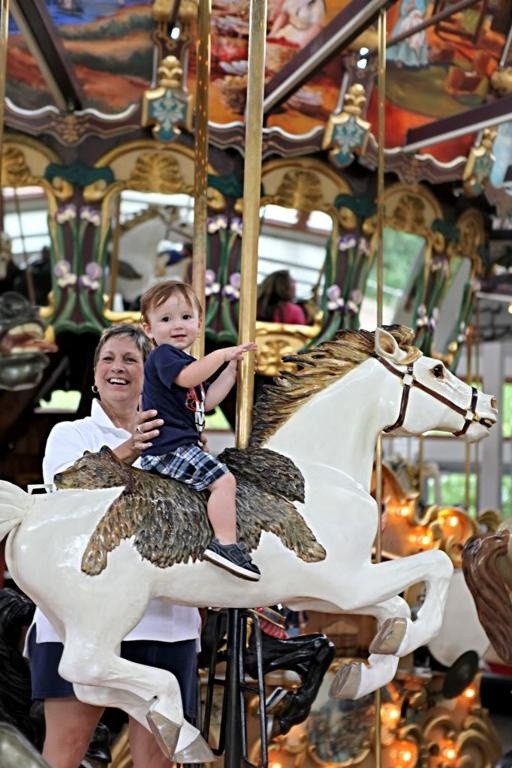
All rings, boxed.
[135,425,143,434]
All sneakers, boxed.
[203,536,262,584]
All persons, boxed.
[139,280,261,582]
[253,269,306,325]
[21,320,203,768]
